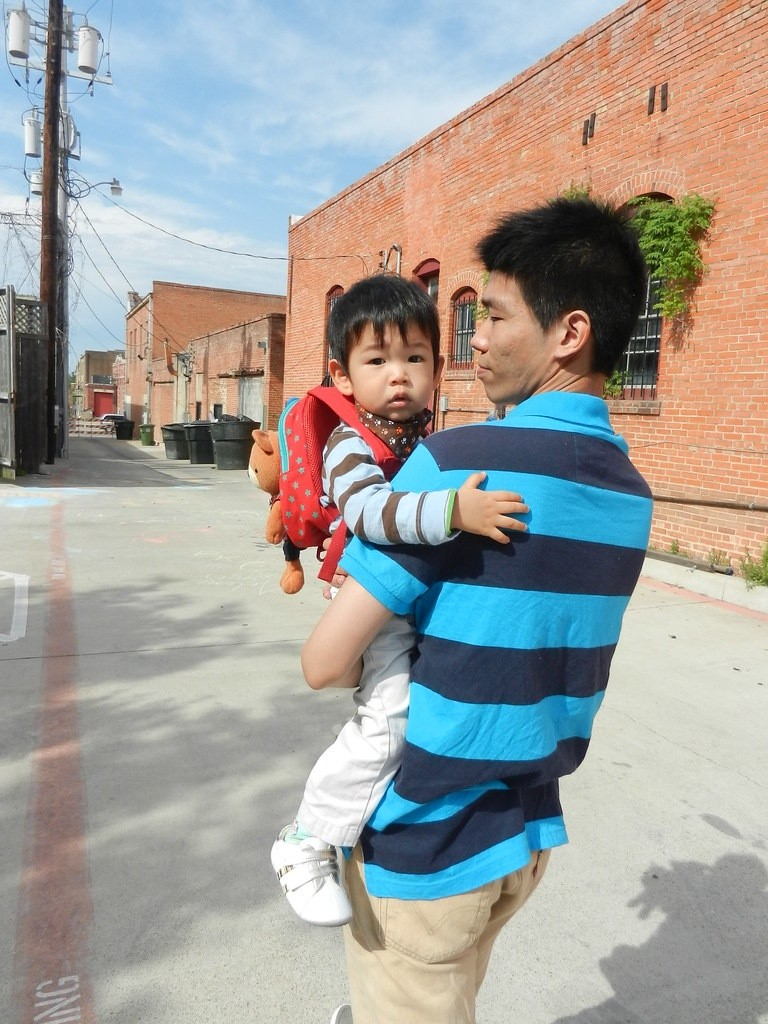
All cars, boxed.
[100,414,123,432]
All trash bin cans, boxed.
[160,422,189,461]
[183,420,216,465]
[210,421,261,471]
[114,420,135,441]
[138,423,156,446]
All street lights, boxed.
[54,10,125,456]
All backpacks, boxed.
[277,386,401,582]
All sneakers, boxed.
[271,824,353,928]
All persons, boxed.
[300,194,660,1022]
[267,277,531,930]
